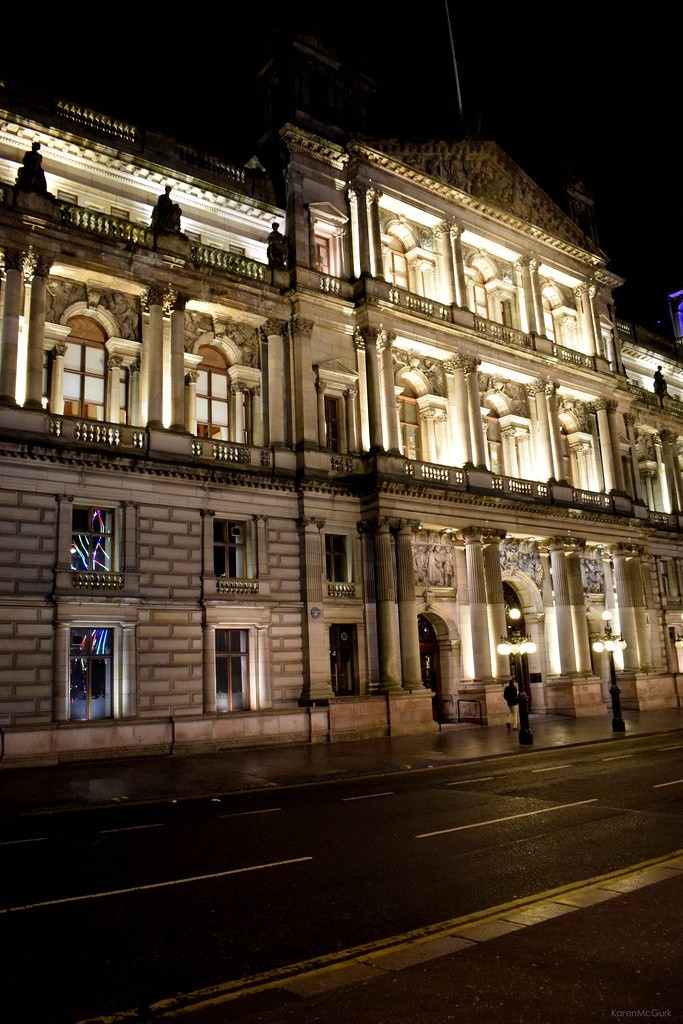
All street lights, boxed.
[591,609,628,732]
[496,607,534,745]
